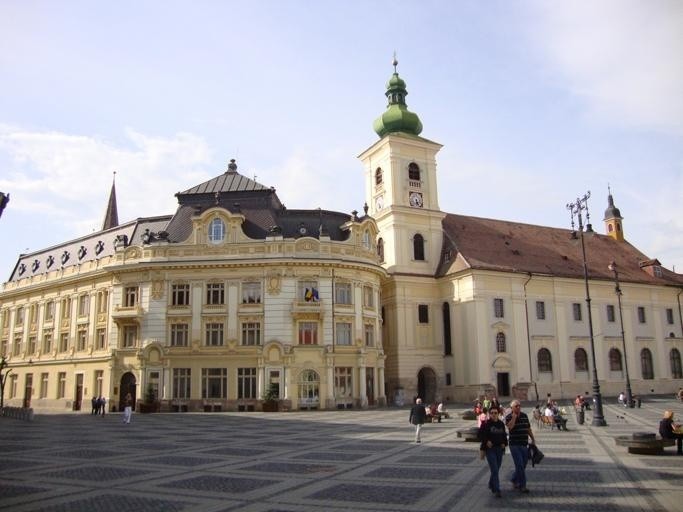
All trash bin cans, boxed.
[635,398,641,408]
[576,408,584,424]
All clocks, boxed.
[409,193,422,208]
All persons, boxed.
[409,387,682,498]
[92,396,106,417]
[123,392,133,424]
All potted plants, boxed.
[139,384,157,414]
[262,383,278,412]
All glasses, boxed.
[489,412,497,415]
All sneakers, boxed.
[492,489,500,497]
[518,486,529,493]
[511,479,518,489]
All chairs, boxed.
[530,411,565,431]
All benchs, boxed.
[614,435,676,455]
[424,412,484,441]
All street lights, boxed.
[608,260,635,408]
[566,190,607,426]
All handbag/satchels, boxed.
[479,450,484,460]
